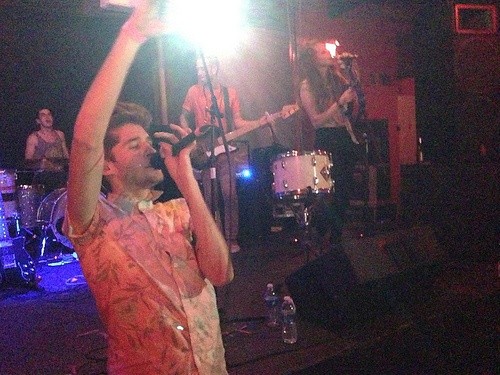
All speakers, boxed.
[284,229,432,330]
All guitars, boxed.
[189,104,299,170]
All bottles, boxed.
[263,285,277,327]
[280,296,297,344]
[417,137,424,162]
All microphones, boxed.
[150,125,211,170]
[334,53,358,61]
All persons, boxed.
[61,0,234,375]
[299,38,359,246]
[180,52,273,252]
[25,107,70,170]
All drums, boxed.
[3,200,18,218]
[18,184,46,226]
[271,150,337,202]
[37,187,106,249]
[0,168,18,194]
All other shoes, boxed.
[227,239,241,254]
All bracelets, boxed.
[337,100,343,108]
